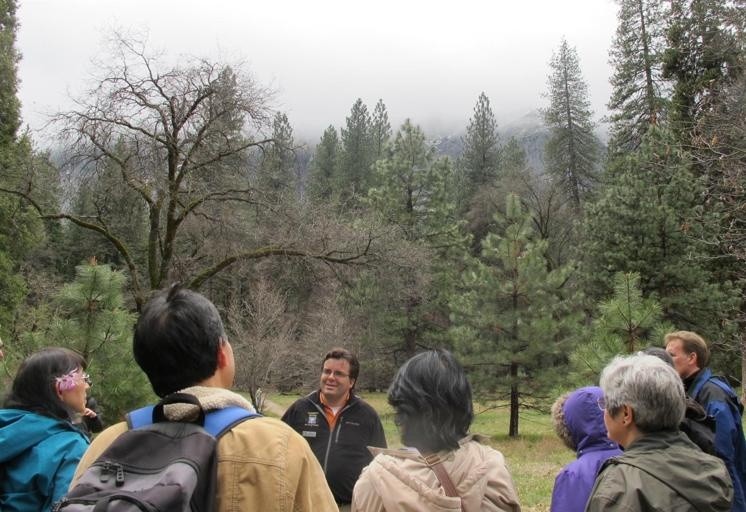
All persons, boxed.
[68,283,341,512]
[350,347,522,512]
[279,347,388,511]
[585,349,734,511]
[646,332,745,511]
[549,384,626,511]
[71,408,104,439]
[1,347,92,511]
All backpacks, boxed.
[48,393,265,512]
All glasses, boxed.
[597,396,610,411]
